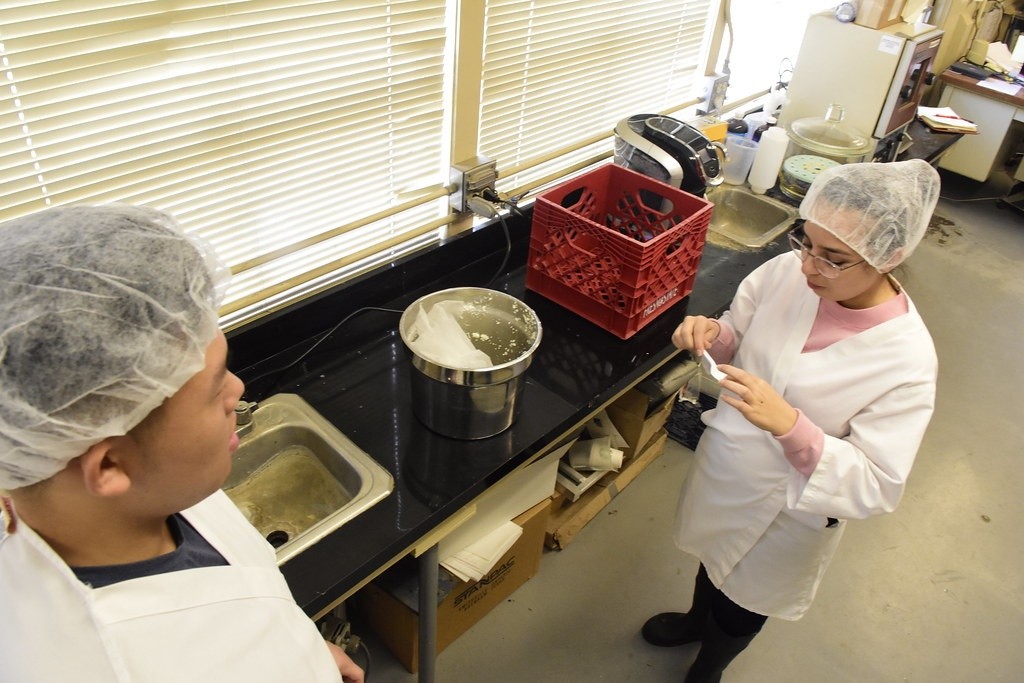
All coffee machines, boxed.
[613,113,724,261]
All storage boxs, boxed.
[438,436,580,564]
[855,0,907,30]
[523,162,716,340]
[544,427,670,551]
[354,499,556,676]
[605,383,688,460]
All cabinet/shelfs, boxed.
[936,85,1016,183]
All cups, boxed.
[723,135,759,186]
[834,0,858,22]
[569,434,624,473]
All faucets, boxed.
[711,141,730,163]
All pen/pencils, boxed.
[934,114,957,119]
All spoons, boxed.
[702,348,730,381]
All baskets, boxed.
[525,162,714,339]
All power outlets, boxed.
[699,72,729,114]
[449,155,500,214]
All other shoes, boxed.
[642,613,701,647]
[684,659,722,683]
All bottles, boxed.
[747,125,789,195]
[751,116,778,142]
[724,109,749,137]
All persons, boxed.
[641,158,942,683]
[0,205,365,683]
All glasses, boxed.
[787,222,865,279]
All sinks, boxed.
[703,182,797,248]
[219,394,394,567]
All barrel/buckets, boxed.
[398,286,543,441]
